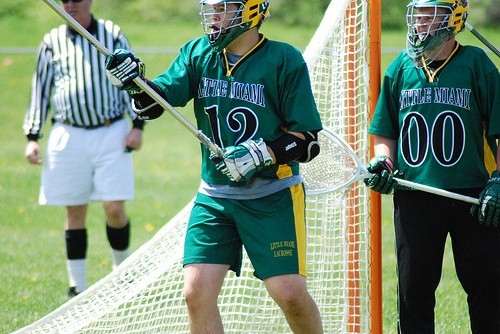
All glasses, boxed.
[60,0,84,3]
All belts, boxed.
[52,114,124,130]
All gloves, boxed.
[364,156,396,194]
[470,170,500,227]
[105,49,145,91]
[209,138,273,182]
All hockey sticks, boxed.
[298,126,482,205]
[42,1,220,153]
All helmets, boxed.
[406,0,469,69]
[199,0,270,52]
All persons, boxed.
[364,1,499,334]
[105,0,321,334]
[23,0,145,299]
